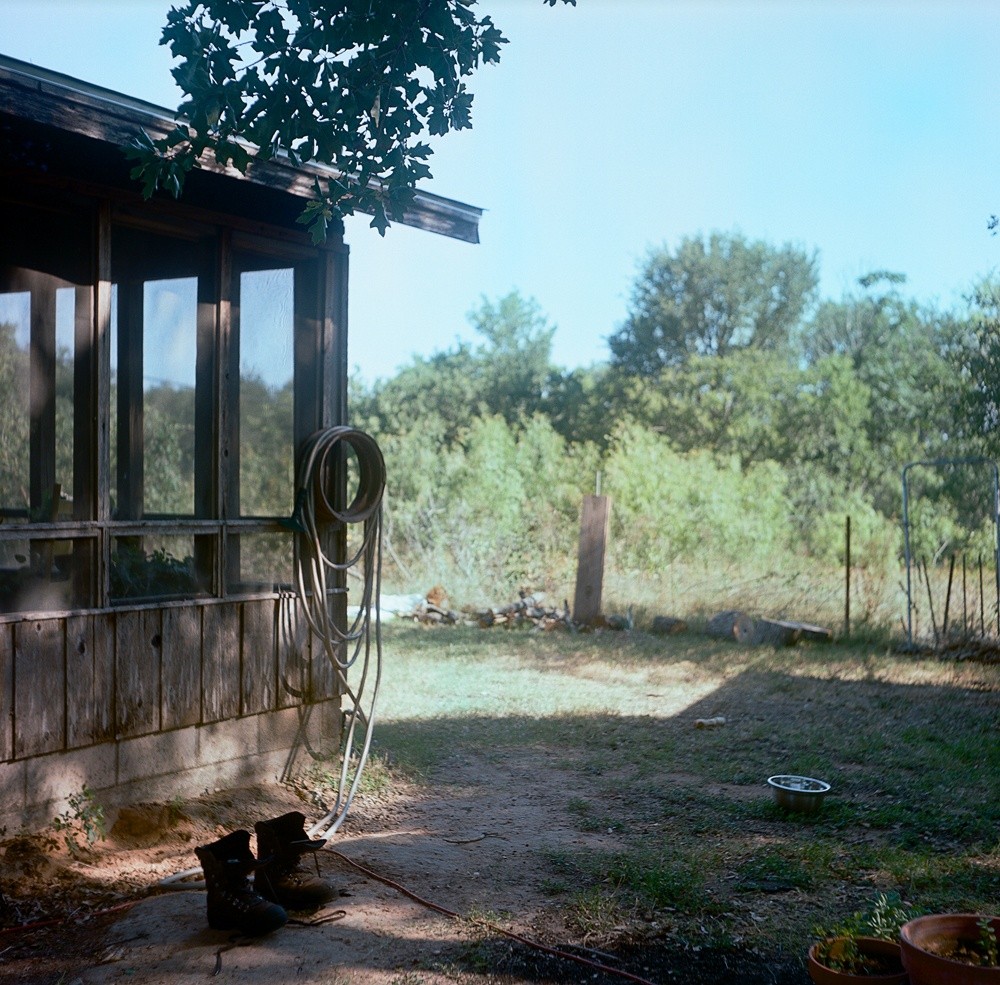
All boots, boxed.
[196,828,286,935]
[253,810,339,909]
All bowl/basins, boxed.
[767,774,831,811]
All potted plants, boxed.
[807,911,1000,985]
